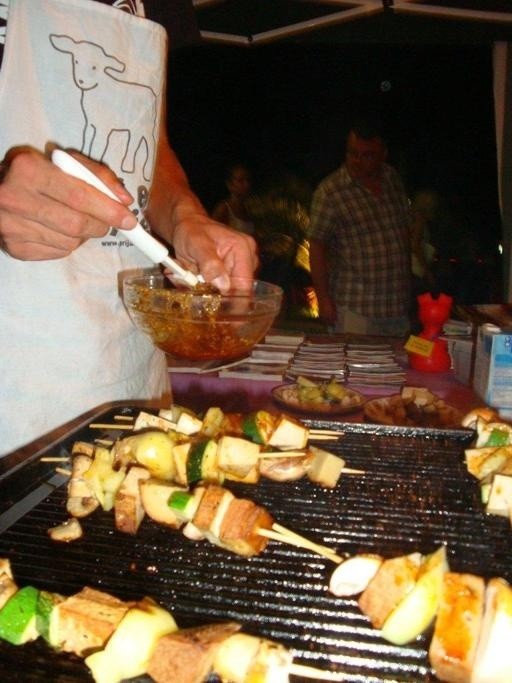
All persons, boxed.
[309,122,504,337]
[215,163,320,320]
[0,0,259,460]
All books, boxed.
[168,327,407,384]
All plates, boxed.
[274,376,464,428]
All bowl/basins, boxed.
[121,267,285,362]
